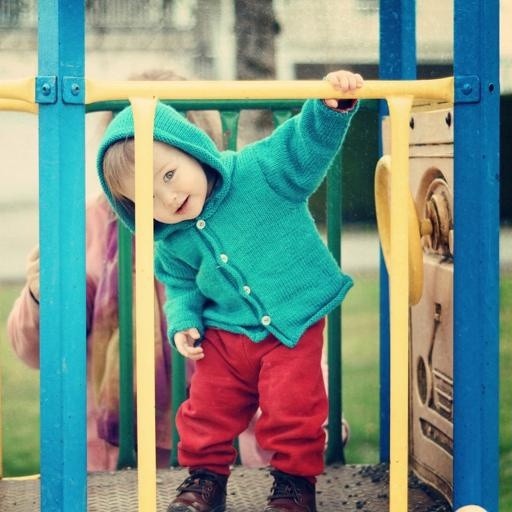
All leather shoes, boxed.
[167,468,228,512]
[262,469,317,512]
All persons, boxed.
[94,70,365,512]
[6,70,352,473]
